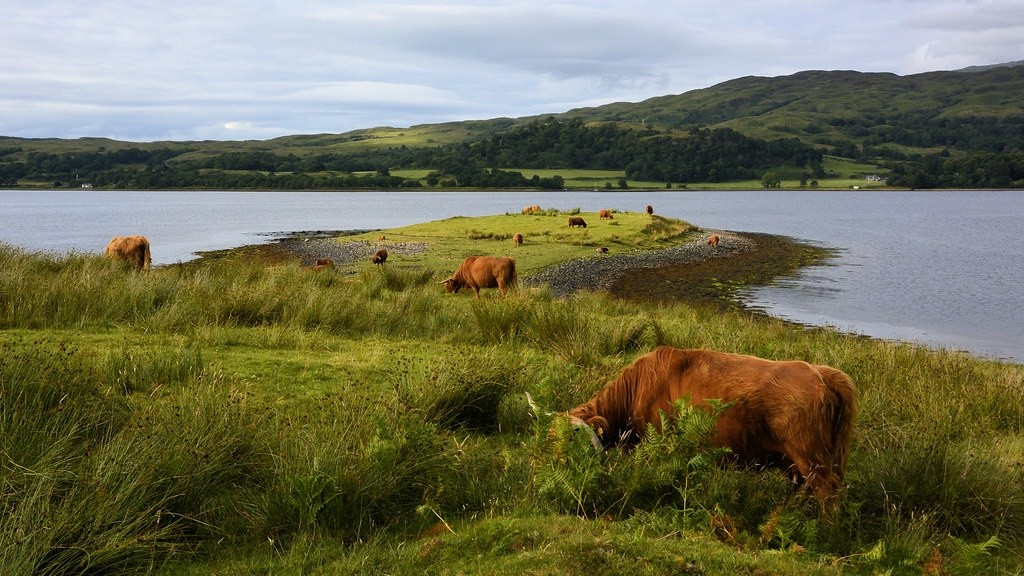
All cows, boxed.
[525,344,860,545]
[599,209,613,220]
[316,258,335,266]
[105,235,152,274]
[523,205,587,229]
[595,247,609,253]
[513,232,523,246]
[646,205,654,215]
[438,255,517,301]
[370,249,387,266]
[379,235,385,241]
[707,235,719,247]
